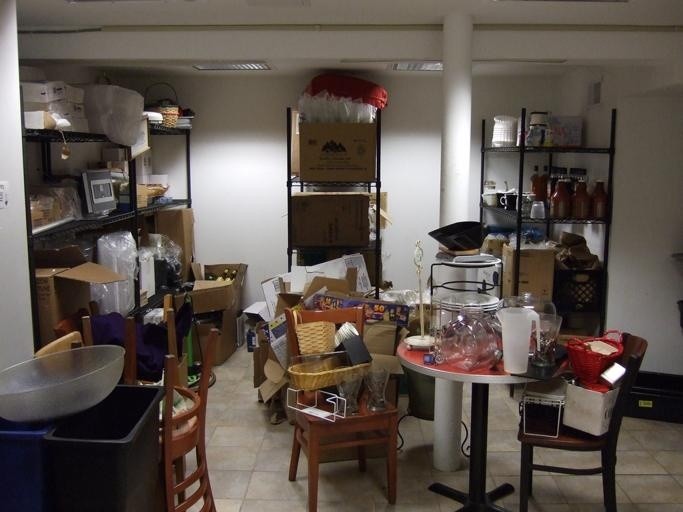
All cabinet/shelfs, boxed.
[287,107,381,300]
[22,129,191,354]
[480,108,616,337]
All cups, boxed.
[482,193,546,219]
[334,366,363,415]
[366,367,390,411]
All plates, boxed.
[403,335,435,349]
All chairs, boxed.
[83,294,218,511]
[518,332,648,512]
[283,306,398,512]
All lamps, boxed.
[50,112,72,161]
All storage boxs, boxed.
[44,385,165,511]
[501,242,555,302]
[102,148,169,184]
[33,245,129,348]
[154,208,194,283]
[21,81,89,132]
[292,192,370,247]
[291,111,300,176]
[300,110,377,182]
[0,418,52,512]
[190,263,247,314]
[481,238,510,258]
[192,296,241,366]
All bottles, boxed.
[217,268,229,282]
[591,180,608,217]
[541,164,549,198]
[572,168,592,219]
[225,269,238,282]
[549,166,572,218]
[531,165,540,198]
[207,272,215,280]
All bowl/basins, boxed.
[1,344,127,423]
[335,322,359,346]
